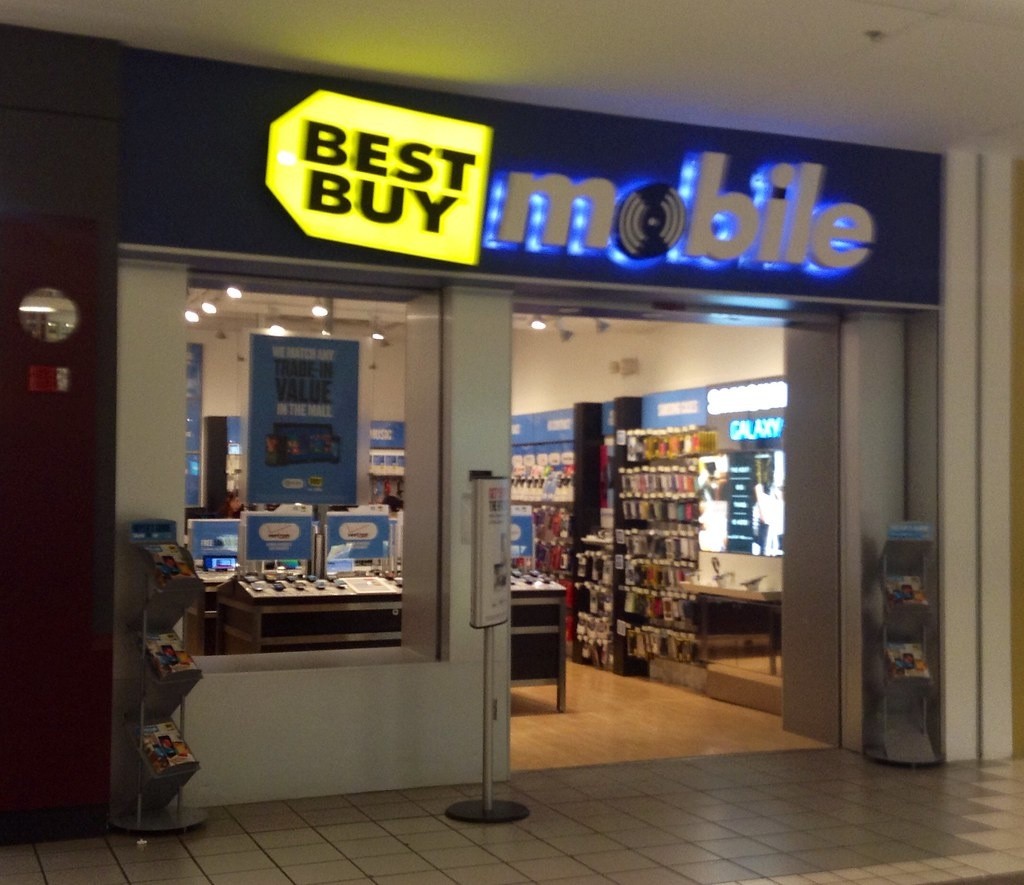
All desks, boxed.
[682,582,782,676]
[215,573,569,714]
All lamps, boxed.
[371,328,386,340]
[554,320,573,344]
[531,314,547,331]
[185,307,200,323]
[201,298,220,315]
[311,297,329,318]
[594,317,611,333]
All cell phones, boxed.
[244,573,345,592]
[509,570,550,585]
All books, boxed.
[133,544,200,776]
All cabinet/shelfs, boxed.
[108,518,209,836]
[510,439,575,578]
[574,550,614,646]
[619,424,716,641]
[863,517,946,769]
